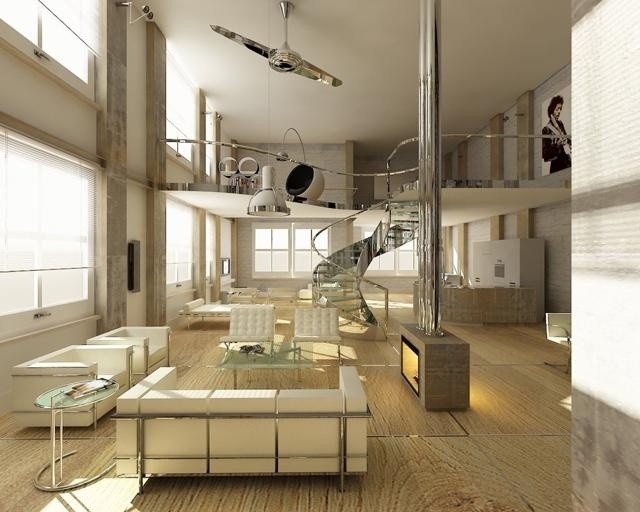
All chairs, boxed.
[542,311,572,375]
[8,343,138,432]
[178,282,315,332]
[285,164,327,205]
[219,307,277,360]
[84,325,173,379]
[292,306,343,364]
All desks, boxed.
[30,376,123,495]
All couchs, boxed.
[108,365,376,498]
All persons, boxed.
[542,95,572,173]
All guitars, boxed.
[551,114,571,160]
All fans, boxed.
[206,1,344,91]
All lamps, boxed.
[274,125,307,166]
[244,3,294,218]
